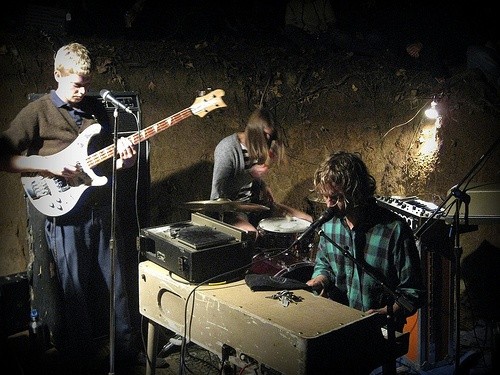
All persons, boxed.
[0,43,169,368]
[305,151,425,331]
[211,107,314,242]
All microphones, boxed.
[100,88,132,114]
[292,207,334,246]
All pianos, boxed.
[138,255,411,375]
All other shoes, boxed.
[131,352,167,368]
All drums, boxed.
[257,216,316,267]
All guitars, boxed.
[20,87,228,218]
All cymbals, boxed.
[181,199,270,212]
